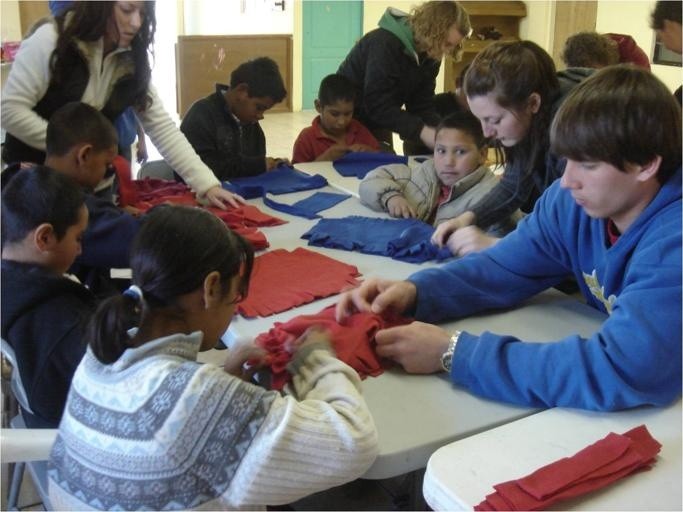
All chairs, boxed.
[136,159,176,181]
[0,339,50,511]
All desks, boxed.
[215,159,605,480]
[422,403,682,512]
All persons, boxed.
[293,74,379,159]
[358,112,523,229]
[50,204,380,511]
[116,102,150,171]
[1,167,86,430]
[554,32,620,91]
[334,61,682,409]
[650,0,681,99]
[175,55,286,181]
[337,0,471,148]
[404,66,474,156]
[39,98,167,279]
[0,0,247,211]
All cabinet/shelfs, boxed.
[443,0,526,94]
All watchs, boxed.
[442,329,465,377]
